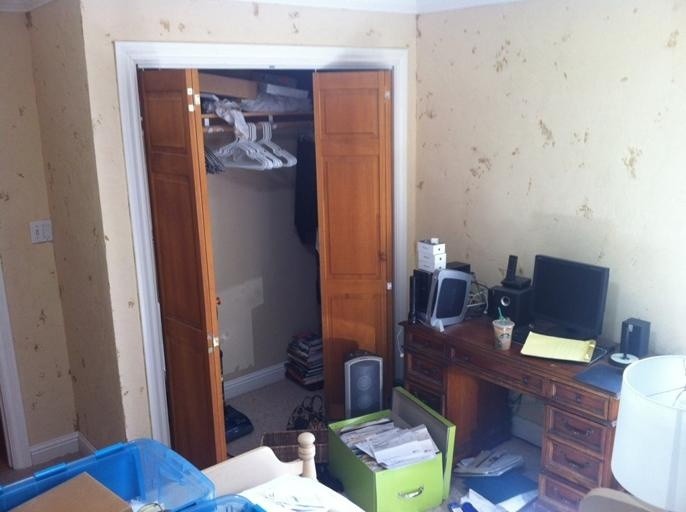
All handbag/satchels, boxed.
[286,393,328,431]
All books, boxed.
[259,328,539,511]
[573,362,623,395]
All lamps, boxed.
[610,354,686,512]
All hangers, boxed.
[203,121,299,174]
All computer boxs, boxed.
[414,261,470,324]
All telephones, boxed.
[500,255,531,290]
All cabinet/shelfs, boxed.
[137,66,396,471]
[397,318,621,512]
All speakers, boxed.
[342,349,384,418]
[621,318,650,359]
[488,285,533,325]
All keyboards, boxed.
[512,325,607,368]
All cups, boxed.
[492,319,516,350]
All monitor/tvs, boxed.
[529,254,609,341]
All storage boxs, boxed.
[326,385,457,512]
[0,437,217,512]
